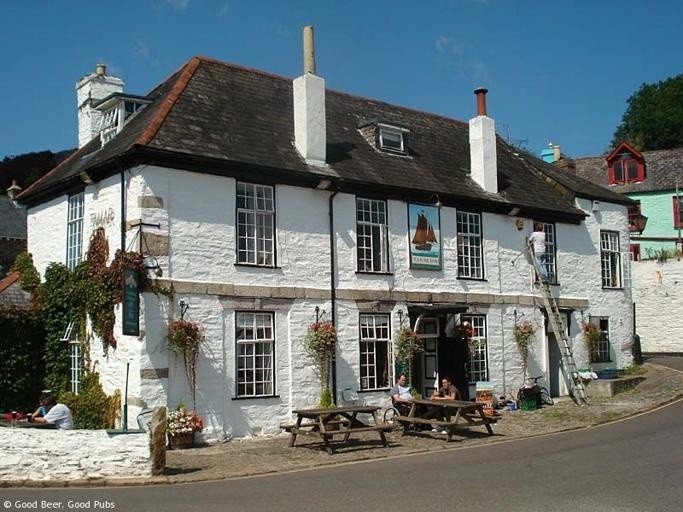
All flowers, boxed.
[513,319,538,375]
[580,321,603,364]
[453,321,485,358]
[303,320,342,388]
[394,326,430,365]
[163,319,205,435]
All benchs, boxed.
[279,419,398,455]
[394,412,502,442]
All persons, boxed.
[31,394,74,430]
[390,374,461,434]
[529,224,549,284]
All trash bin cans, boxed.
[475,381,494,417]
[519,383,537,411]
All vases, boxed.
[167,433,195,449]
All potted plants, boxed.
[314,389,340,431]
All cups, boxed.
[10,419,17,429]
[11,412,17,419]
[26,414,32,422]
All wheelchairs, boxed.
[383,395,401,430]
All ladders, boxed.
[527,239,590,406]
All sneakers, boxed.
[431,426,450,435]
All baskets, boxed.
[520,399,537,410]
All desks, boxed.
[402,398,494,444]
[0,417,56,429]
[287,406,391,455]
